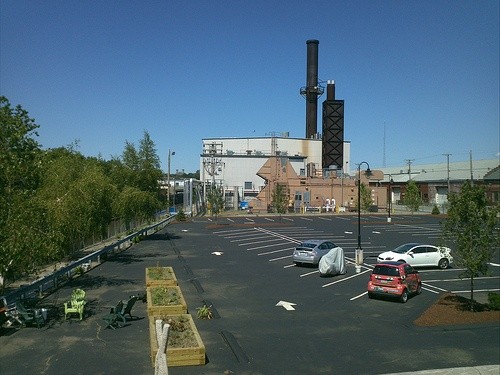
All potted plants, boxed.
[145,267,178,287]
[149,314,205,367]
[147,286,187,316]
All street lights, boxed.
[387,174,394,223]
[353,160,372,264]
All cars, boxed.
[367,260,422,303]
[376,242,454,269]
[292,239,337,266]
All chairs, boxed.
[0,284,59,330]
[64,289,86,320]
[103,297,137,330]
